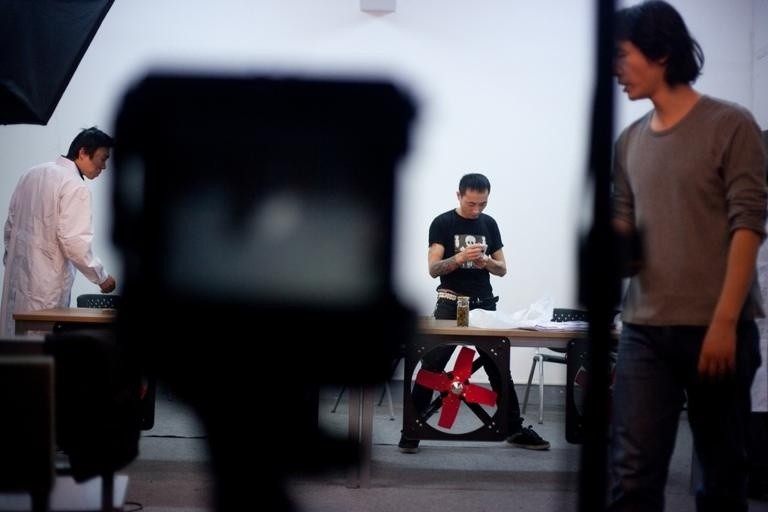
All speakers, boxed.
[0,0,116,127]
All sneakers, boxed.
[507,428,551,451]
[397,429,420,454]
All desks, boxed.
[341,317,625,488]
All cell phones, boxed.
[476,243,488,257]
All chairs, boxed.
[0,292,148,512]
[330,384,396,420]
[522,309,583,425]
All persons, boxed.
[1,121,124,343]
[563,2,767,512]
[396,171,552,460]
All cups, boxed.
[457,300,469,326]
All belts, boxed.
[437,292,500,307]
